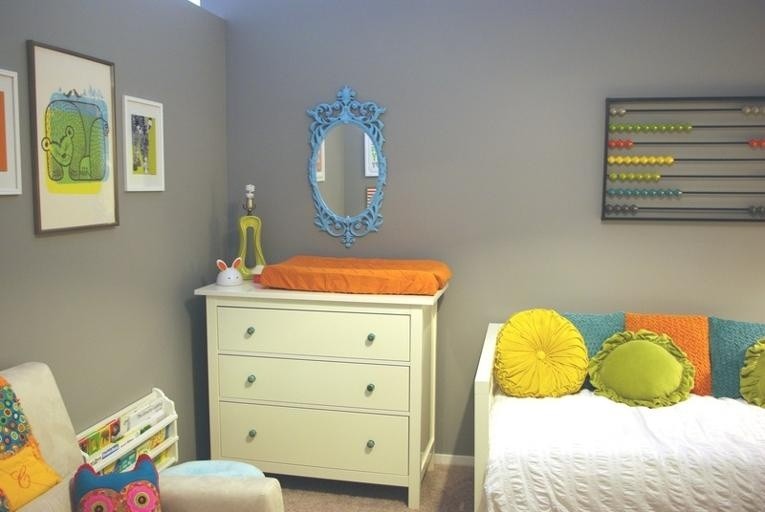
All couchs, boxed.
[469,305,765,512]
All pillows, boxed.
[497,307,765,407]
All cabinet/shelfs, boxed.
[195,277,447,512]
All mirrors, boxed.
[307,83,388,249]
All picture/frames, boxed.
[0,36,164,237]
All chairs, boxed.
[1,360,287,509]
